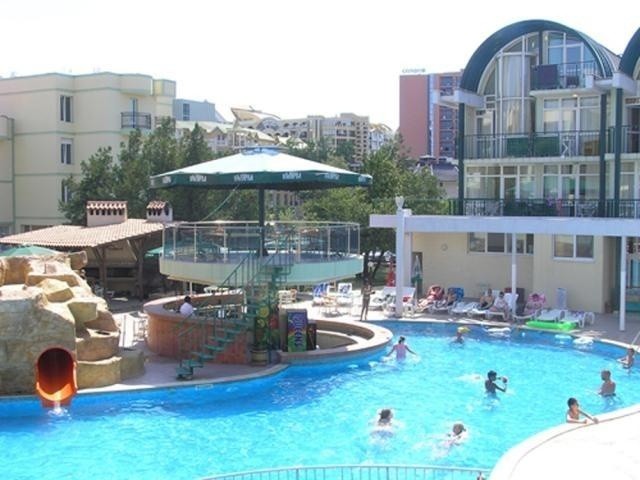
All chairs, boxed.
[203,282,594,331]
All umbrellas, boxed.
[412,255,424,305]
[148,145,374,256]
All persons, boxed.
[448,421,467,446]
[360,277,374,320]
[566,398,598,424]
[417,285,541,322]
[452,331,465,341]
[368,408,397,426]
[180,296,195,316]
[386,335,417,360]
[485,370,509,393]
[618,348,635,370]
[598,370,616,394]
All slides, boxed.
[36,361,78,402]
